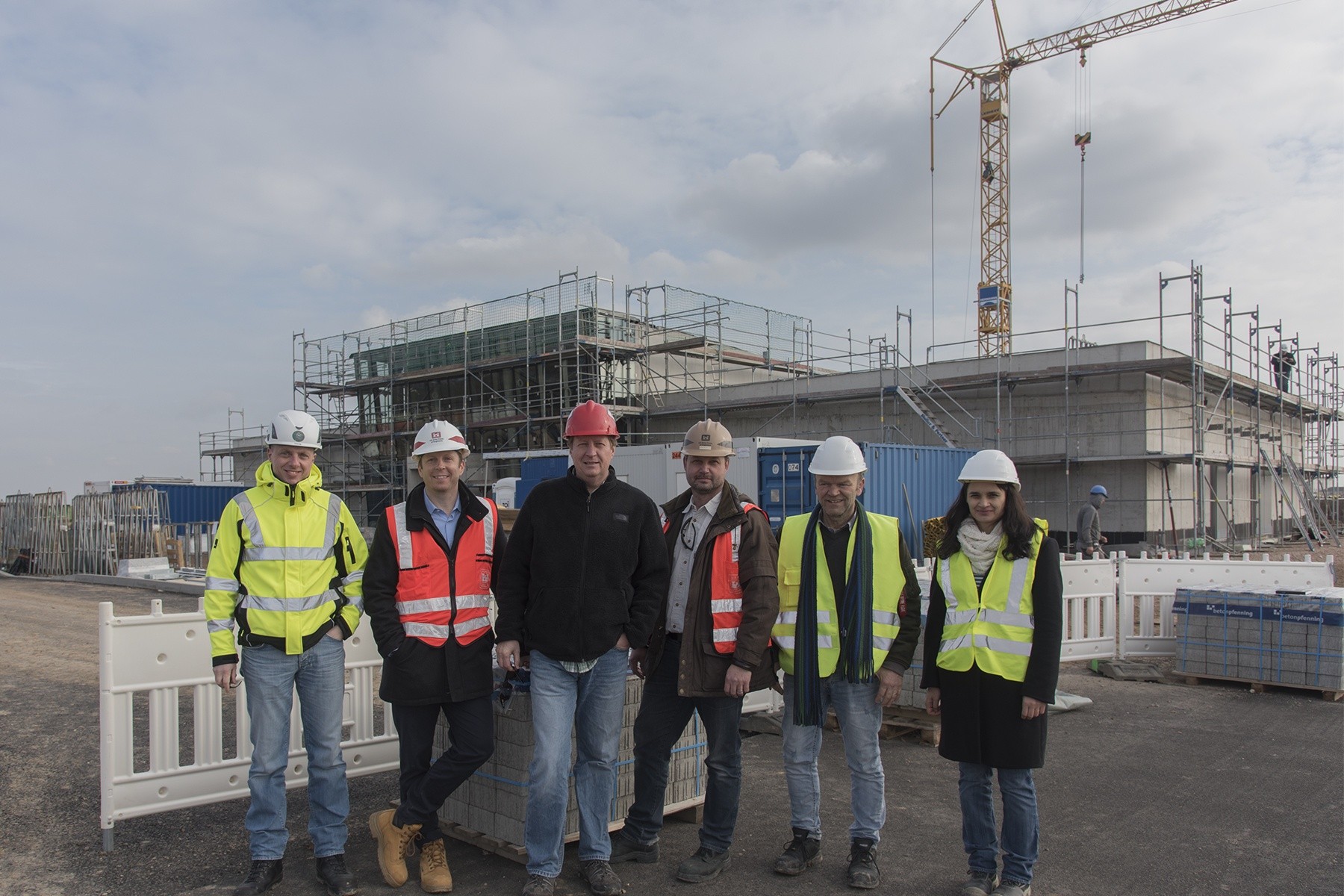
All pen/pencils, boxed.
[510,661,515,667]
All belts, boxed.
[668,633,683,640]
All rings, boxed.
[737,690,742,693]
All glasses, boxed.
[496,660,518,709]
[681,517,696,551]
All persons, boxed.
[361,420,509,893]
[202,410,369,896]
[493,400,670,896]
[609,418,780,885]
[1271,343,1296,394]
[1076,485,1108,559]
[919,450,1063,896]
[768,436,922,888]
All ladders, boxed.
[711,359,723,386]
[1307,422,1319,464]
[636,354,664,407]
[333,397,349,428]
[601,358,620,400]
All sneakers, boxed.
[846,836,881,888]
[607,832,661,864]
[419,838,452,892]
[369,809,422,888]
[961,870,999,896]
[675,846,731,883]
[316,854,358,896]
[990,877,1031,896]
[578,860,622,896]
[522,874,556,896]
[234,857,283,896]
[773,826,823,875]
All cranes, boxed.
[928,0,1240,357]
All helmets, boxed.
[562,399,620,441]
[808,435,868,476]
[956,450,1021,493]
[264,410,322,450]
[1090,485,1108,499]
[412,419,471,461]
[680,418,736,457]
[1280,344,1289,351]
